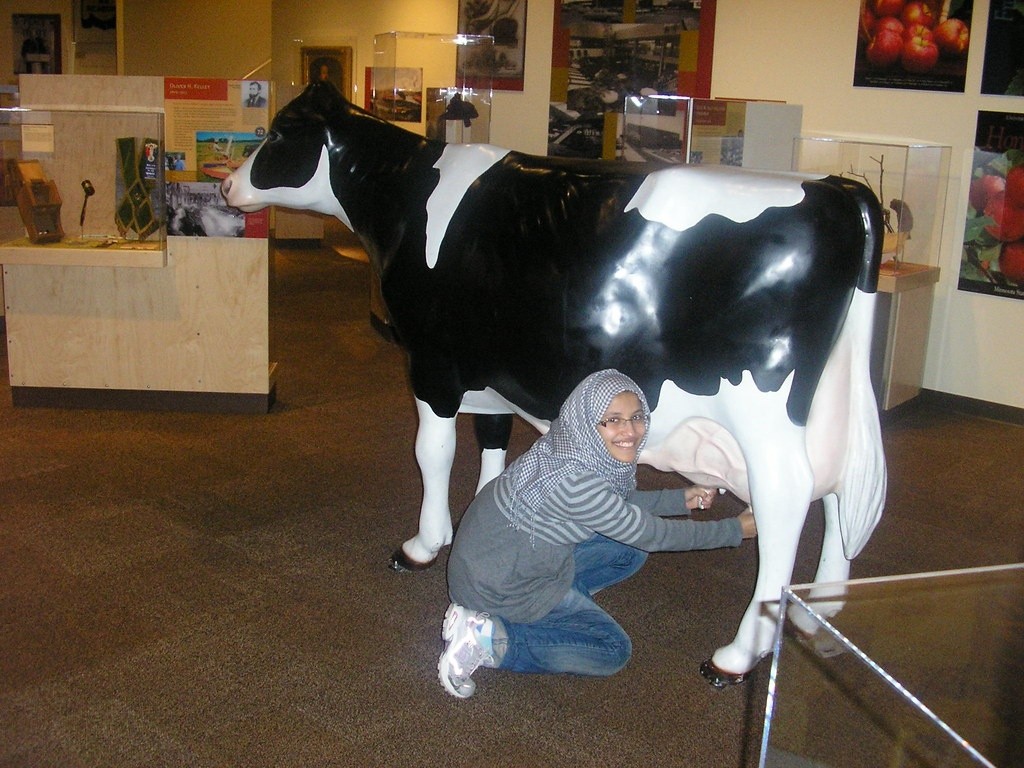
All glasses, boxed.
[598,415,645,427]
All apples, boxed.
[970,166,1024,286]
[858,0,969,72]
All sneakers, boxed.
[438,602,494,698]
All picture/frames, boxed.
[302,45,353,114]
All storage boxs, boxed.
[759,564,1024,768]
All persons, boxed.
[437,368,757,699]
